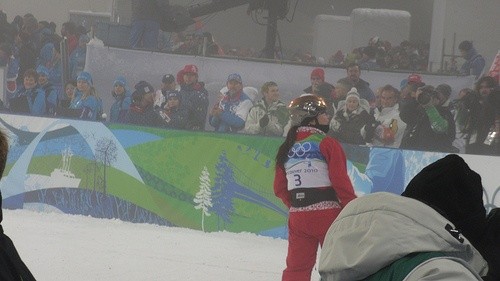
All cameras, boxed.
[418,86,438,104]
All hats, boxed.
[459,39,473,49]
[35,65,49,79]
[400,73,452,95]
[161,73,175,83]
[226,73,243,83]
[135,81,154,94]
[358,97,373,110]
[345,87,360,105]
[310,67,325,80]
[401,153,486,237]
[336,76,353,87]
[112,77,128,91]
[76,72,95,88]
[181,64,198,74]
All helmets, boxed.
[288,92,327,124]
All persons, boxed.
[273,92,357,281]
[316,152,500,281]
[0,11,500,156]
